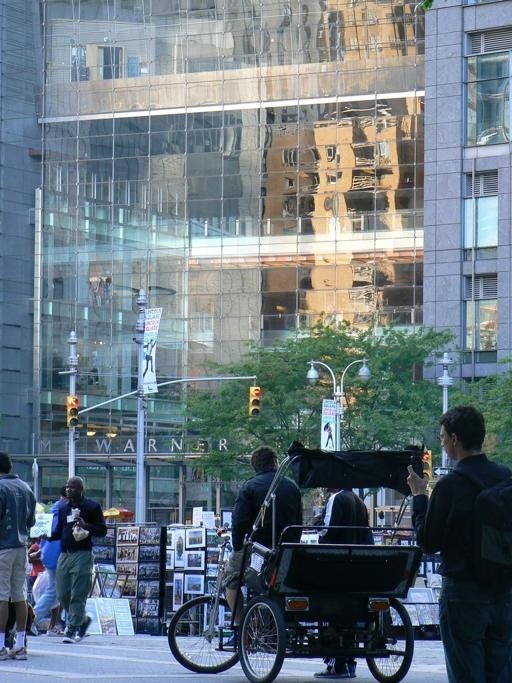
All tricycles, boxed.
[167,439,425,683]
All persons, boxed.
[324,420,335,449]
[142,337,157,378]
[221,444,303,651]
[313,486,375,678]
[405,405,511,682]
[0,451,109,661]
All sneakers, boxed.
[348,661,356,676]
[30,623,38,635]
[0,646,7,659]
[74,615,91,641]
[47,627,64,636]
[314,663,351,677]
[63,626,75,642]
[8,647,28,659]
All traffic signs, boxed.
[431,466,454,477]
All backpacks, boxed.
[453,464,512,585]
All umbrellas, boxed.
[103,507,134,524]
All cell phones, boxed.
[411,455,424,479]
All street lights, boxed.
[434,350,455,465]
[306,357,369,451]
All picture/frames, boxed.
[86,507,240,637]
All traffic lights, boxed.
[248,385,260,416]
[422,450,433,481]
[67,393,79,429]
